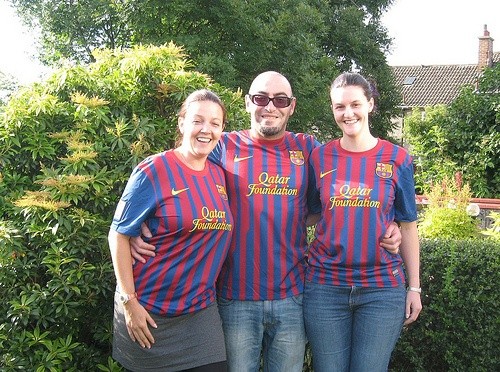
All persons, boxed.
[129,71,402,372]
[305,73,422,372]
[107,89,234,372]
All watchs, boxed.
[407,287,421,294]
[117,293,137,304]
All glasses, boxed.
[247,93,294,108]
